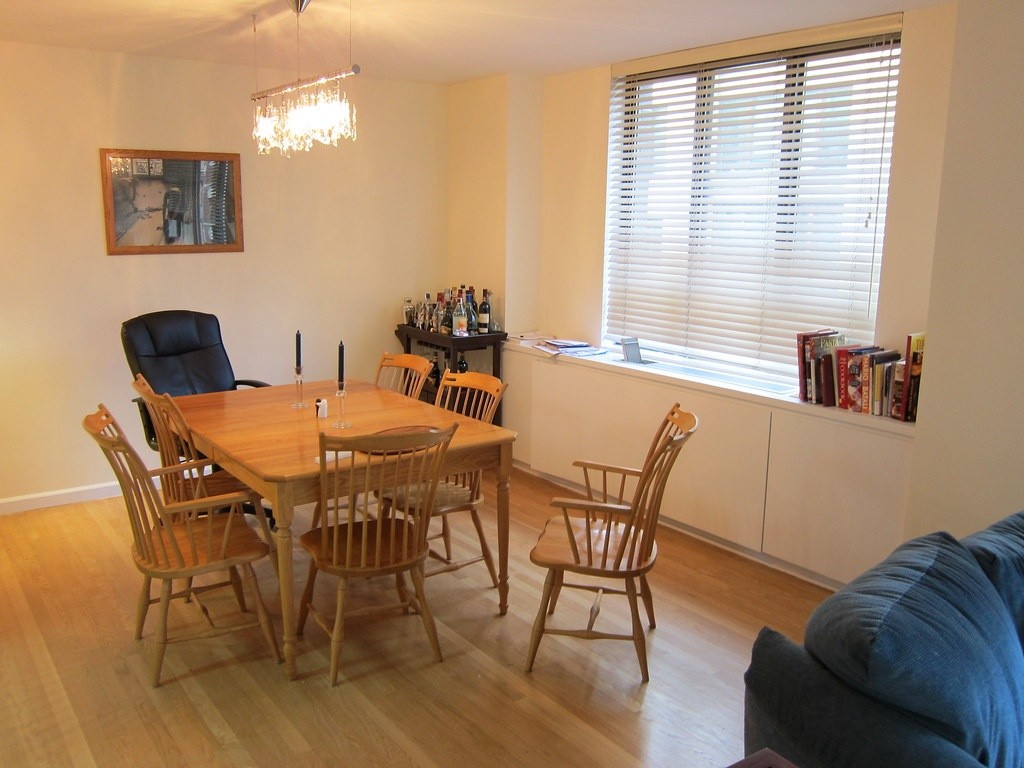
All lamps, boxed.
[252,0,360,159]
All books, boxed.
[797,327,926,423]
[506,333,592,352]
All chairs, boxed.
[311,351,434,581]
[523,401,700,683]
[81,402,282,689]
[120,310,278,531]
[371,368,509,615]
[132,373,279,604]
[297,422,459,688]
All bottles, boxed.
[416,285,490,336]
[456,352,468,391]
[421,351,438,387]
[401,298,415,324]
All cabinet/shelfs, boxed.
[394,323,509,428]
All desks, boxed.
[159,378,518,683]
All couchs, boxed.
[744,512,1024,768]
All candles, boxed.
[337,340,344,390]
[295,330,302,374]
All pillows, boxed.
[959,512,1024,655]
[805,530,1024,768]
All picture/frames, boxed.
[100,148,245,256]
